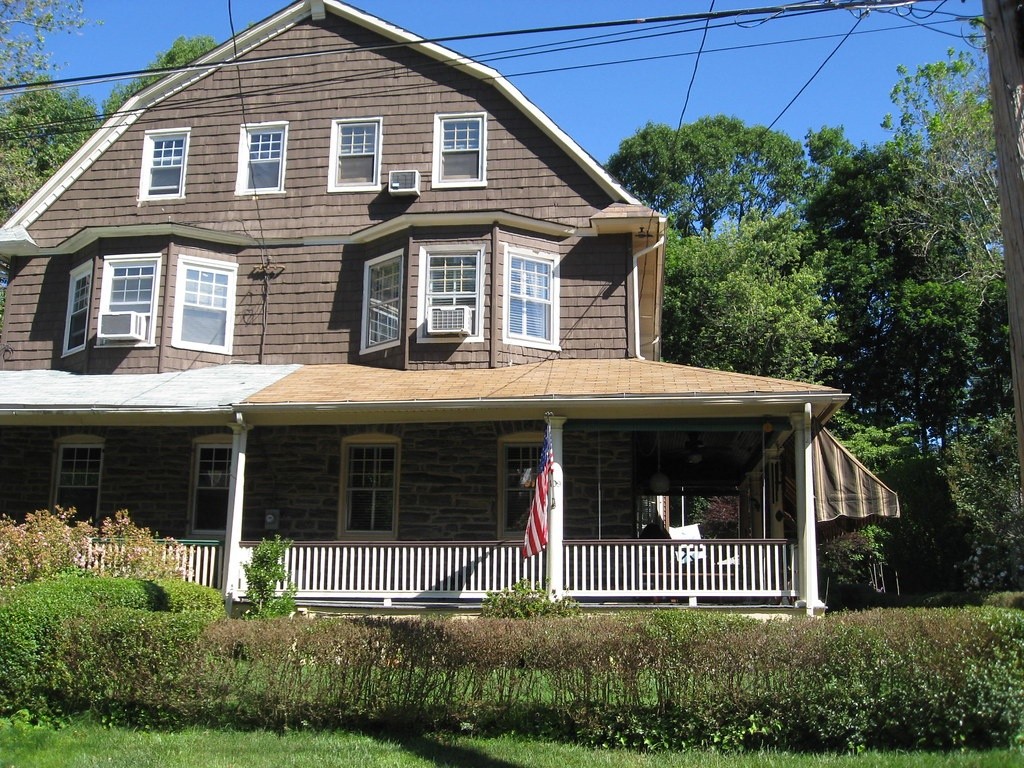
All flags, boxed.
[523,430,553,559]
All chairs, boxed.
[667,523,720,574]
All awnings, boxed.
[811,418,899,522]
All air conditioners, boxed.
[96,312,147,341]
[388,170,422,197]
[427,305,472,336]
